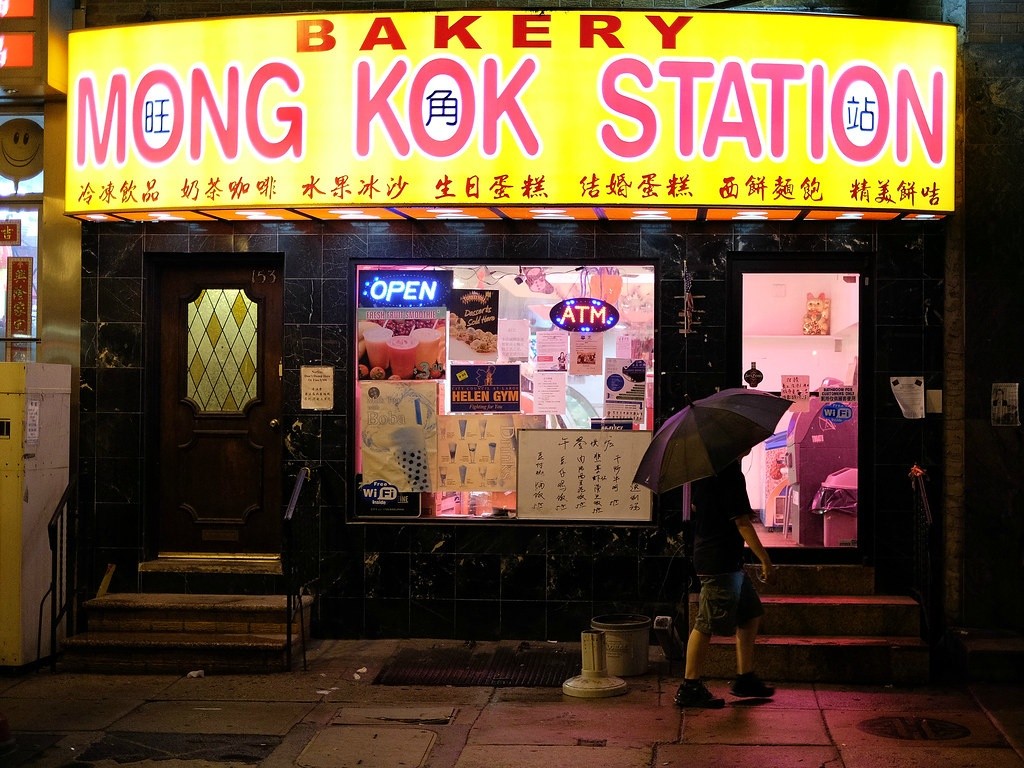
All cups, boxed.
[363,327,393,370]
[386,336,419,379]
[411,329,441,372]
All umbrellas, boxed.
[632,387,796,494]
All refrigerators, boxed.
[759,430,792,527]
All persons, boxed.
[993,389,1007,405]
[672,447,775,709]
[558,352,567,370]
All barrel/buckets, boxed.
[589,613,651,677]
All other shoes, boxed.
[676,680,725,709]
[729,671,775,697]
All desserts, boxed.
[449,313,497,354]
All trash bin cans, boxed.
[815,466,858,546]
